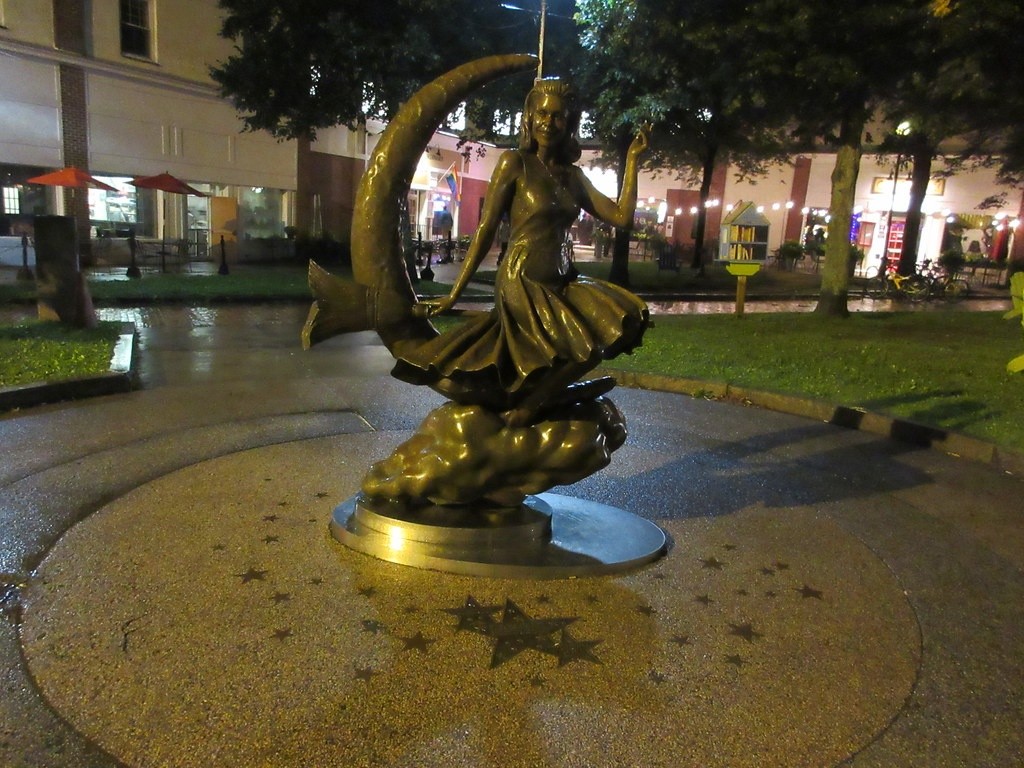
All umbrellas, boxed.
[989,219,1008,288]
[123,170,206,274]
[27,168,120,272]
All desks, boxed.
[137,239,179,273]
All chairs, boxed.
[26,235,195,276]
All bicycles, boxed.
[866,258,970,303]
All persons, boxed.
[438,205,453,240]
[391,76,655,426]
